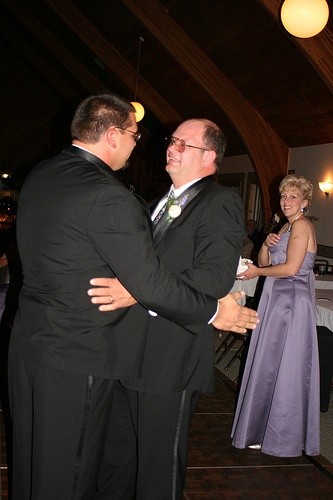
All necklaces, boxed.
[282,213,304,233]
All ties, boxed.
[151,190,174,231]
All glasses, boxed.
[113,127,140,141]
[165,138,209,153]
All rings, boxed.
[111,296,114,303]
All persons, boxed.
[235,173,321,457]
[7,94,260,500]
[87,119,244,500]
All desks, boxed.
[314,255,333,413]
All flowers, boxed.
[164,191,189,223]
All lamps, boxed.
[318,181,330,199]
